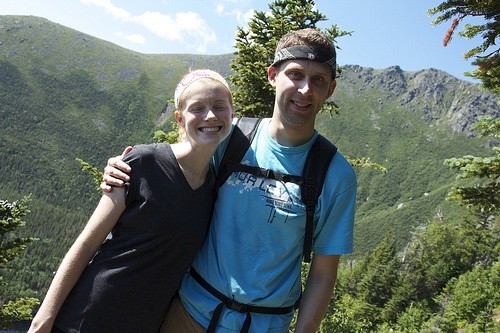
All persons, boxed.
[98,28,357,333]
[26,69,235,333]
